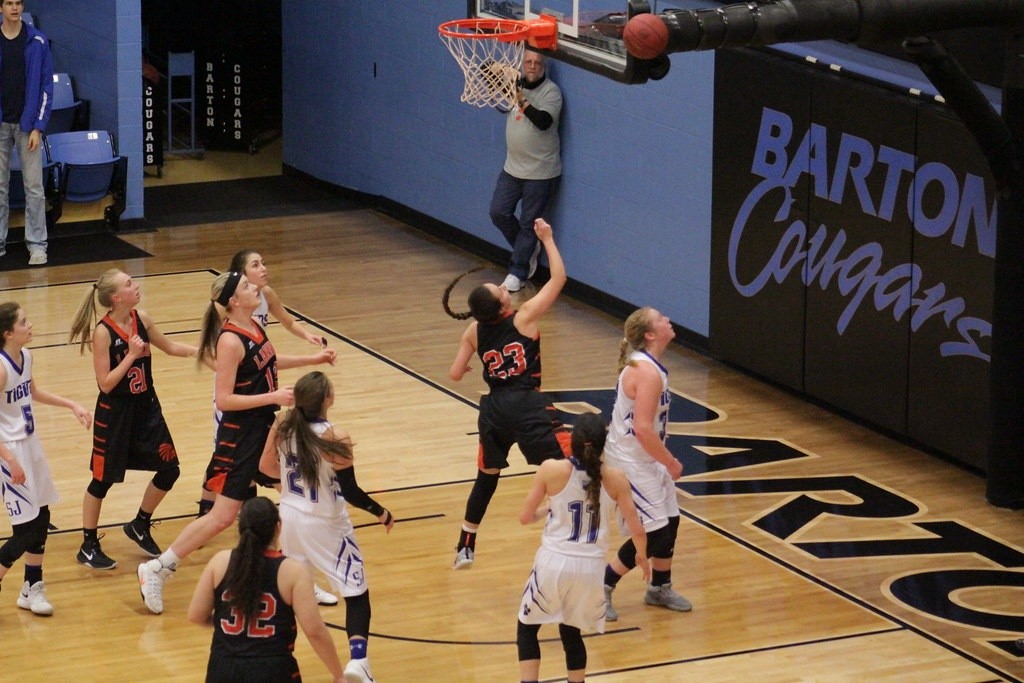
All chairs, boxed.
[11,73,128,236]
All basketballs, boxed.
[622,13,670,60]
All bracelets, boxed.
[384,512,391,525]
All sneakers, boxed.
[501,273,525,292]
[645,583,692,612]
[17,580,53,615]
[527,239,542,280]
[344,657,376,683]
[313,583,337,605]
[603,583,618,621]
[453,543,474,570]
[124,519,162,556]
[137,556,176,614]
[28,248,47,265]
[77,533,118,570]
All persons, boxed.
[597,307,691,620]
[257,370,395,683]
[441,216,573,571]
[68,267,216,569]
[138,271,337,616]
[188,496,347,683]
[212,249,326,450]
[0,301,93,617]
[516,412,651,683]
[0,0,54,265]
[481,49,564,292]
